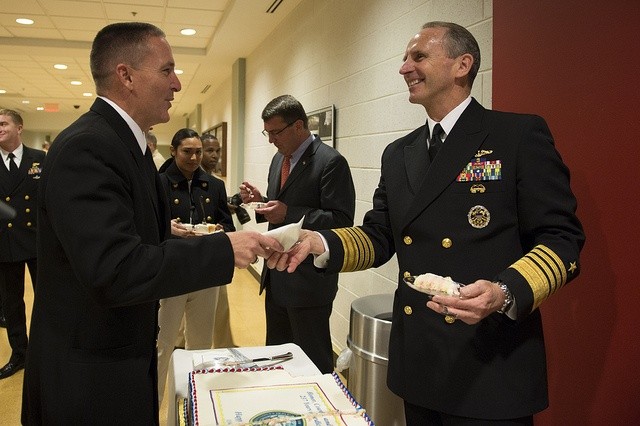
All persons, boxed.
[240,95,356,374]
[145,132,166,172]
[159,129,236,426]
[21,22,284,426]
[266,22,586,426]
[41,141,50,153]
[0,109,46,379]
[175,135,239,350]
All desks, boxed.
[159,342,324,426]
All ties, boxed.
[7,151,18,174]
[427,123,444,163]
[280,155,290,189]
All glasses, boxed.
[262,123,295,140]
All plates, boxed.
[239,202,269,211]
[403,275,467,303]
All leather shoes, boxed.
[0,360,27,380]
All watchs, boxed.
[492,281,514,315]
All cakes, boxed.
[413,273,461,298]
[194,223,217,234]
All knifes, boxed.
[223,351,293,366]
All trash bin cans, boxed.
[347,295,405,426]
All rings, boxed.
[251,256,259,264]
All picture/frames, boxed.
[301,104,335,148]
[202,121,228,178]
